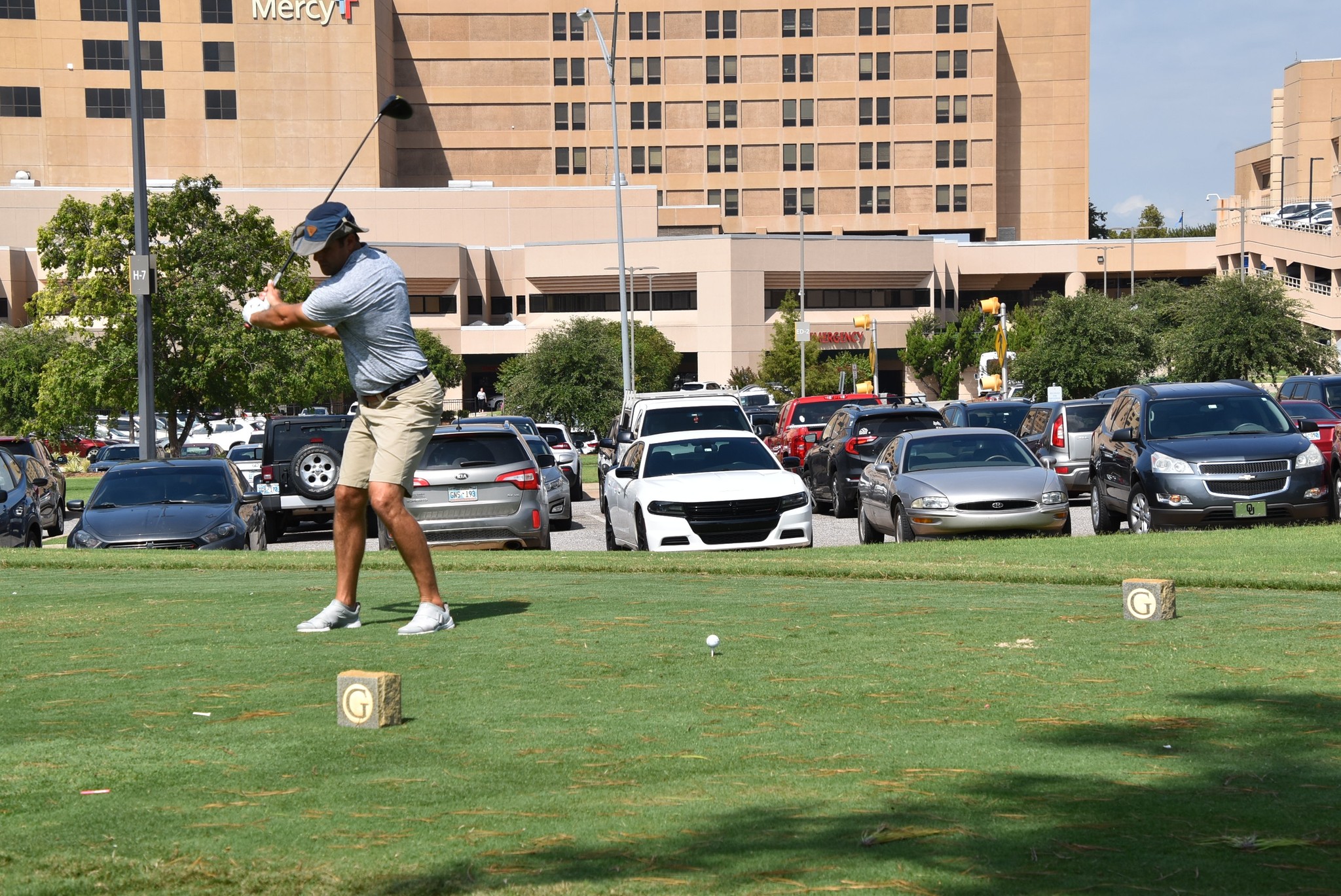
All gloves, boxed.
[242,297,269,326]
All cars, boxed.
[0,351,1341,550]
[1260,201,1332,235]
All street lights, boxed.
[1281,156,1295,223]
[1108,226,1159,295]
[1308,157,1325,226]
[1211,206,1273,286]
[794,210,811,398]
[576,0,632,392]
[1085,246,1125,296]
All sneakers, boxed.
[398,602,455,635]
[296,599,361,632]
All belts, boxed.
[363,366,431,403]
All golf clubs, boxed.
[244,94,414,329]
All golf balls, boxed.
[706,634,719,648]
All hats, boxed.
[290,202,369,256]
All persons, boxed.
[242,202,455,635]
[477,388,486,413]
[501,403,504,413]
